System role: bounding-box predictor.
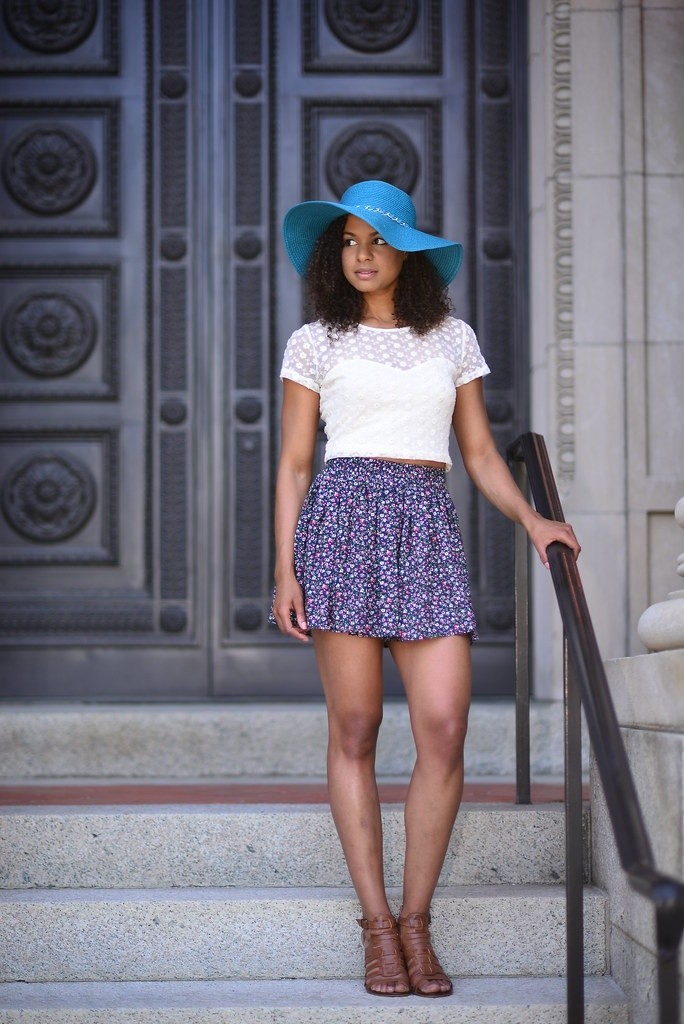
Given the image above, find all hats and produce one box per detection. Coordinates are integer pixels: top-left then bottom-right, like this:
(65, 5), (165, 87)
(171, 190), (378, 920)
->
(281, 179), (464, 289)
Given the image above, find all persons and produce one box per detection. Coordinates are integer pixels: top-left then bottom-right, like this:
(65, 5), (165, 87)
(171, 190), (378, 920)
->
(265, 180), (584, 1000)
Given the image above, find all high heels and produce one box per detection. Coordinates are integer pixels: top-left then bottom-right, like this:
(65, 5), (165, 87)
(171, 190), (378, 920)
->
(396, 906), (453, 998)
(355, 913), (411, 996)
